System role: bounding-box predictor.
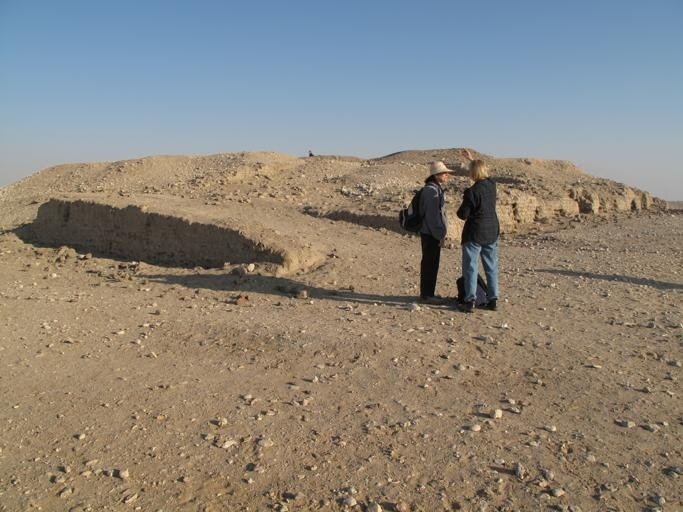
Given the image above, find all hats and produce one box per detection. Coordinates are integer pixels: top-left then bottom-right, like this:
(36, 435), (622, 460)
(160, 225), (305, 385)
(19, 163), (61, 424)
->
(424, 160), (456, 183)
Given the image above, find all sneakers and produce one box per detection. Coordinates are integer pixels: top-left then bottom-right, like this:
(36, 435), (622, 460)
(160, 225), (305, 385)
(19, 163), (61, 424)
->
(420, 296), (442, 305)
(485, 300), (496, 309)
(461, 300), (476, 312)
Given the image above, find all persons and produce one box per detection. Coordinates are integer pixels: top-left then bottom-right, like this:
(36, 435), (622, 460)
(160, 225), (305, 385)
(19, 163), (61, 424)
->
(418, 160), (459, 305)
(454, 146), (501, 313)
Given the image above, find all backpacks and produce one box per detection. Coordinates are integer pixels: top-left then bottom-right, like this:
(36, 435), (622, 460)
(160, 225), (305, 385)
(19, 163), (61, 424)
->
(456, 272), (488, 306)
(399, 184), (440, 233)
